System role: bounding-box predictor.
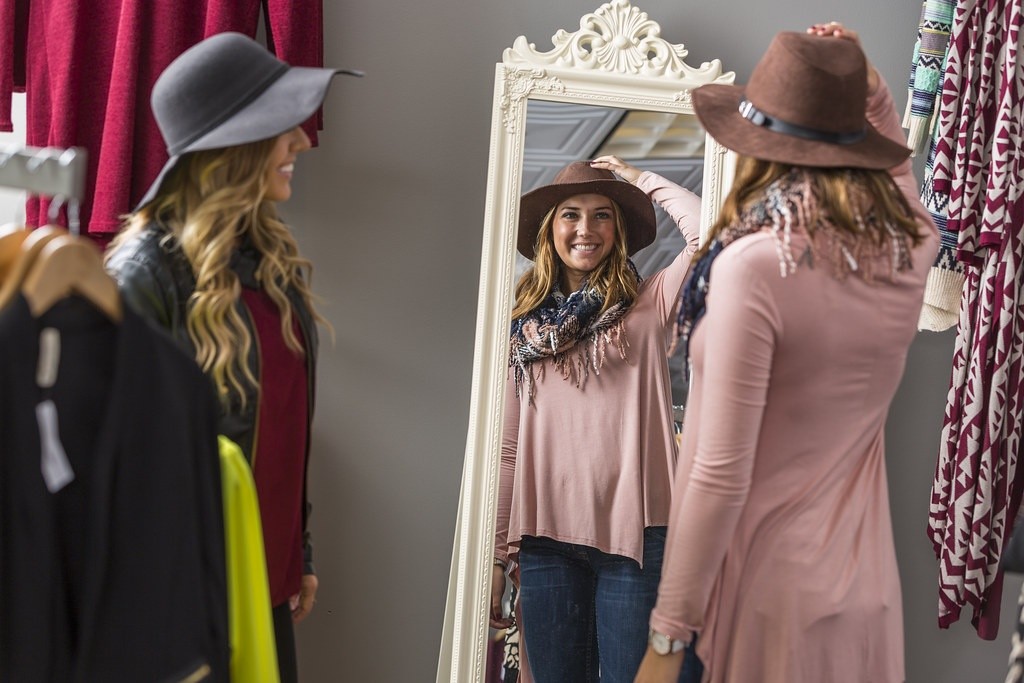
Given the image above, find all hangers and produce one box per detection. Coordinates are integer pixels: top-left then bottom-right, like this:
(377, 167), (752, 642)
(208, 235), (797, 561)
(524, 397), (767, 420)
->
(0, 147), (202, 393)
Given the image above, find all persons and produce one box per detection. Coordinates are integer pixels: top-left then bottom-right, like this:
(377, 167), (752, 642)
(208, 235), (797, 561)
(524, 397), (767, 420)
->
(102, 32), (366, 683)
(488, 155), (701, 683)
(633, 21), (940, 683)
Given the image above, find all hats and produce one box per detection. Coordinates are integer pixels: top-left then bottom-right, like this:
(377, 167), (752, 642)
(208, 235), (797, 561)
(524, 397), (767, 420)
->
(691, 30), (916, 171)
(516, 162), (659, 263)
(131, 32), (364, 210)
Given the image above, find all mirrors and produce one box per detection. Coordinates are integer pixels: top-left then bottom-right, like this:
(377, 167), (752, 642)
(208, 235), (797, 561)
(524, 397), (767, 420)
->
(434, 0), (737, 683)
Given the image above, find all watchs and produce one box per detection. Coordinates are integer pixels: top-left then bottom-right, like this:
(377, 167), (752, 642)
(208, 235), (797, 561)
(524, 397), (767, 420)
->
(647, 630), (684, 655)
(494, 559), (507, 570)
